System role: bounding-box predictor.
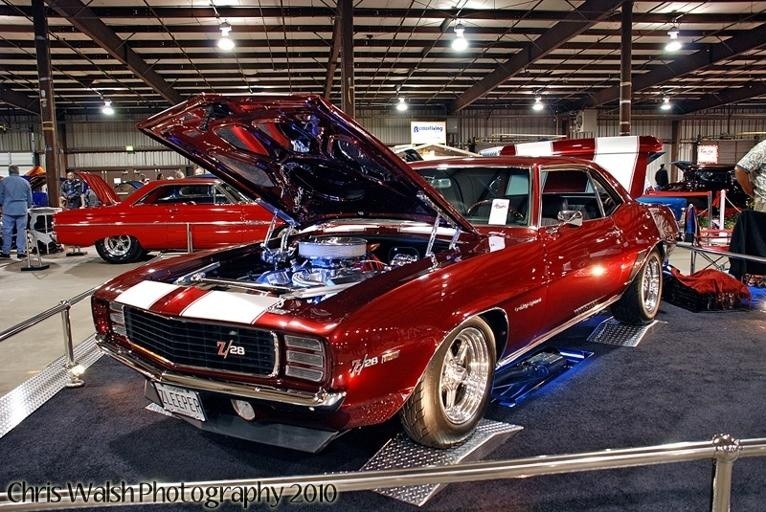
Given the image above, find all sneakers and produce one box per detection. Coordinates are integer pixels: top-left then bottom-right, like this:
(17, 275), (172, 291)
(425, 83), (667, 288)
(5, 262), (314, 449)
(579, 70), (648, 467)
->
(17, 254), (27, 258)
(0, 254), (10, 258)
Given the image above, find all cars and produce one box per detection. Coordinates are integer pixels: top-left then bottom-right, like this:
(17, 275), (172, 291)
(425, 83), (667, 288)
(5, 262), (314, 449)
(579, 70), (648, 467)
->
(660, 159), (753, 209)
(89, 91), (680, 451)
(51, 170), (301, 265)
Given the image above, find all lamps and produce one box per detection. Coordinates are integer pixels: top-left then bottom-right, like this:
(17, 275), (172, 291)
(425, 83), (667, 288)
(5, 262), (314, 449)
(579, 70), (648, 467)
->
(532, 96), (544, 112)
(664, 26), (682, 51)
(215, 22), (236, 50)
(101, 98), (114, 115)
(452, 24), (469, 49)
(660, 96), (671, 110)
(396, 97), (409, 112)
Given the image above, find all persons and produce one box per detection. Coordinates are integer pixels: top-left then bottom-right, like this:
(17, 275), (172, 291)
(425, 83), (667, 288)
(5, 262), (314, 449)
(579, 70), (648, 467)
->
(156, 174), (164, 181)
(733, 137), (766, 216)
(174, 169), (185, 180)
(139, 173), (149, 185)
(655, 163), (669, 188)
(60, 172), (87, 210)
(0, 164), (33, 260)
